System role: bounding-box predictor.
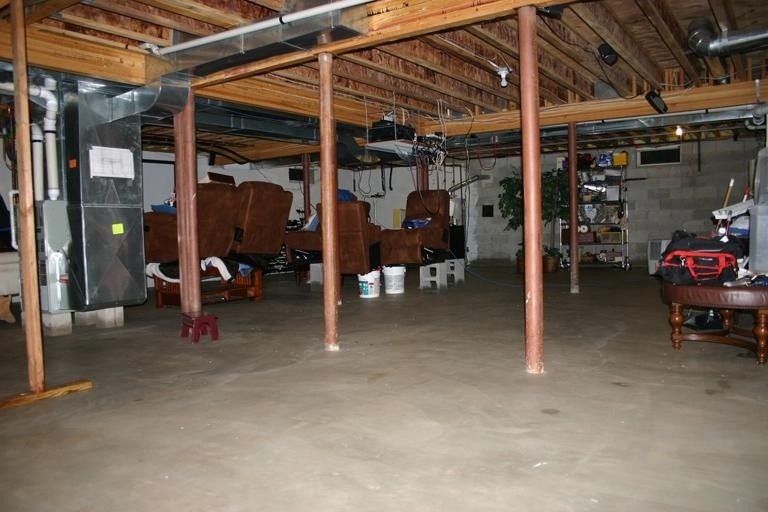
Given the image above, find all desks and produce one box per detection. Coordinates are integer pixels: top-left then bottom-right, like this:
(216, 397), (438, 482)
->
(662, 282), (768, 366)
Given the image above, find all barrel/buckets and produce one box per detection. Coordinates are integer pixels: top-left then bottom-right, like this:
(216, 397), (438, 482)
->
(381, 264), (406, 295)
(357, 269), (383, 298)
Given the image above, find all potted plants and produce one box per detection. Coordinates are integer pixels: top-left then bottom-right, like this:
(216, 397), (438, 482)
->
(497, 169), (582, 274)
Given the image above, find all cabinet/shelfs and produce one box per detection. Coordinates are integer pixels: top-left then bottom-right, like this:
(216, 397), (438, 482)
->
(560, 164), (632, 271)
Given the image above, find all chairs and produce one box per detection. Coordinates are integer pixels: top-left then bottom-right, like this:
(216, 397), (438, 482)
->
(146, 180), (238, 265)
(233, 180), (293, 259)
(374, 187), (450, 267)
(318, 199), (374, 274)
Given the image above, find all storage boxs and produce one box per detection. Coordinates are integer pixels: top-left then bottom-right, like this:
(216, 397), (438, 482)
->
(613, 153), (629, 166)
(579, 231), (626, 244)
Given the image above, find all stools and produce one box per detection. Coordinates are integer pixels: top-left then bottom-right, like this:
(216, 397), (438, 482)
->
(181, 310), (219, 344)
(419, 258), (465, 289)
